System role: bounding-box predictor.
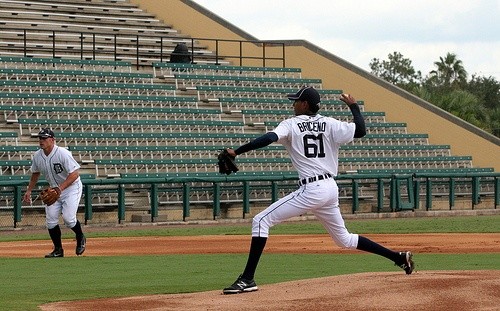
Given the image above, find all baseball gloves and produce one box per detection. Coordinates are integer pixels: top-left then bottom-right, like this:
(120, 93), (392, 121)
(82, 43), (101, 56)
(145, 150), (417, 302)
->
(217, 149), (240, 175)
(40, 187), (62, 207)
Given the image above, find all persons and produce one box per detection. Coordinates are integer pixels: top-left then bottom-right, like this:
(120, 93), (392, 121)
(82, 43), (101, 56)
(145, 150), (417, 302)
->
(23, 129), (86, 257)
(221, 87), (415, 294)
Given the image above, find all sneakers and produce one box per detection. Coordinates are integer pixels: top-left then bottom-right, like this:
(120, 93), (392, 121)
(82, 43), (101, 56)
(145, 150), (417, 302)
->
(75, 236), (86, 255)
(398, 251), (414, 274)
(44, 248), (63, 258)
(223, 273), (258, 293)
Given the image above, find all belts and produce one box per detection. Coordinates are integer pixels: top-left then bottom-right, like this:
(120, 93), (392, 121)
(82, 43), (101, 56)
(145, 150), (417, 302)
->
(299, 173), (332, 186)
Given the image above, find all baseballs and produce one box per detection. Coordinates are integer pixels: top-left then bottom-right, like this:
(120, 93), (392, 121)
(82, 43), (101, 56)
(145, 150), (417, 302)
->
(340, 94), (348, 99)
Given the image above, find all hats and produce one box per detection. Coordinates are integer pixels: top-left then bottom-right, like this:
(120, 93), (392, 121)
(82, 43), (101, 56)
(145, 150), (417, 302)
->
(31, 129), (54, 138)
(288, 87), (320, 103)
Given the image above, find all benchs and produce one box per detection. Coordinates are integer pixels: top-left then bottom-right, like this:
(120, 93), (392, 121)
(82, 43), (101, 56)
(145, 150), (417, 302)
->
(1, 0), (499, 205)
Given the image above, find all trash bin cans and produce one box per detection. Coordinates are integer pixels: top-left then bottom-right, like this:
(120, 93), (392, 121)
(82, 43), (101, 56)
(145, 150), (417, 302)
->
(169, 43), (192, 72)
